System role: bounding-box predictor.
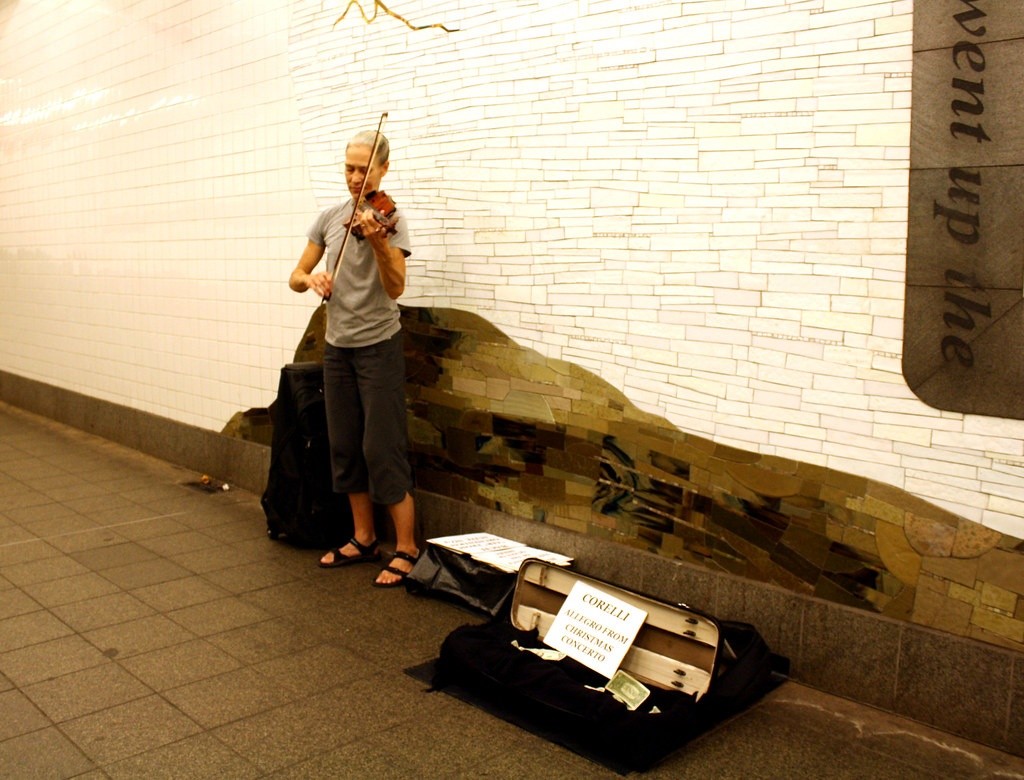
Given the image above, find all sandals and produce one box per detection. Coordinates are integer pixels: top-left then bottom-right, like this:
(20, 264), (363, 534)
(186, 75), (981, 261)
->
(318, 538), (381, 567)
(373, 550), (419, 587)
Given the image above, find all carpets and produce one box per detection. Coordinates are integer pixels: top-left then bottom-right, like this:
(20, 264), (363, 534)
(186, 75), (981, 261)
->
(404, 657), (787, 776)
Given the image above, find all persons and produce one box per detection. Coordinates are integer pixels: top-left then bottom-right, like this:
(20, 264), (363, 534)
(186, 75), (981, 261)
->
(287, 130), (423, 585)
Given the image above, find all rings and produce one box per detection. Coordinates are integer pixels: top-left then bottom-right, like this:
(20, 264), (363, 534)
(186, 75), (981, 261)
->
(361, 224), (366, 229)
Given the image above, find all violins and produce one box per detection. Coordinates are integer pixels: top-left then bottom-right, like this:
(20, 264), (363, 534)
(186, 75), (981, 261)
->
(343, 190), (401, 240)
